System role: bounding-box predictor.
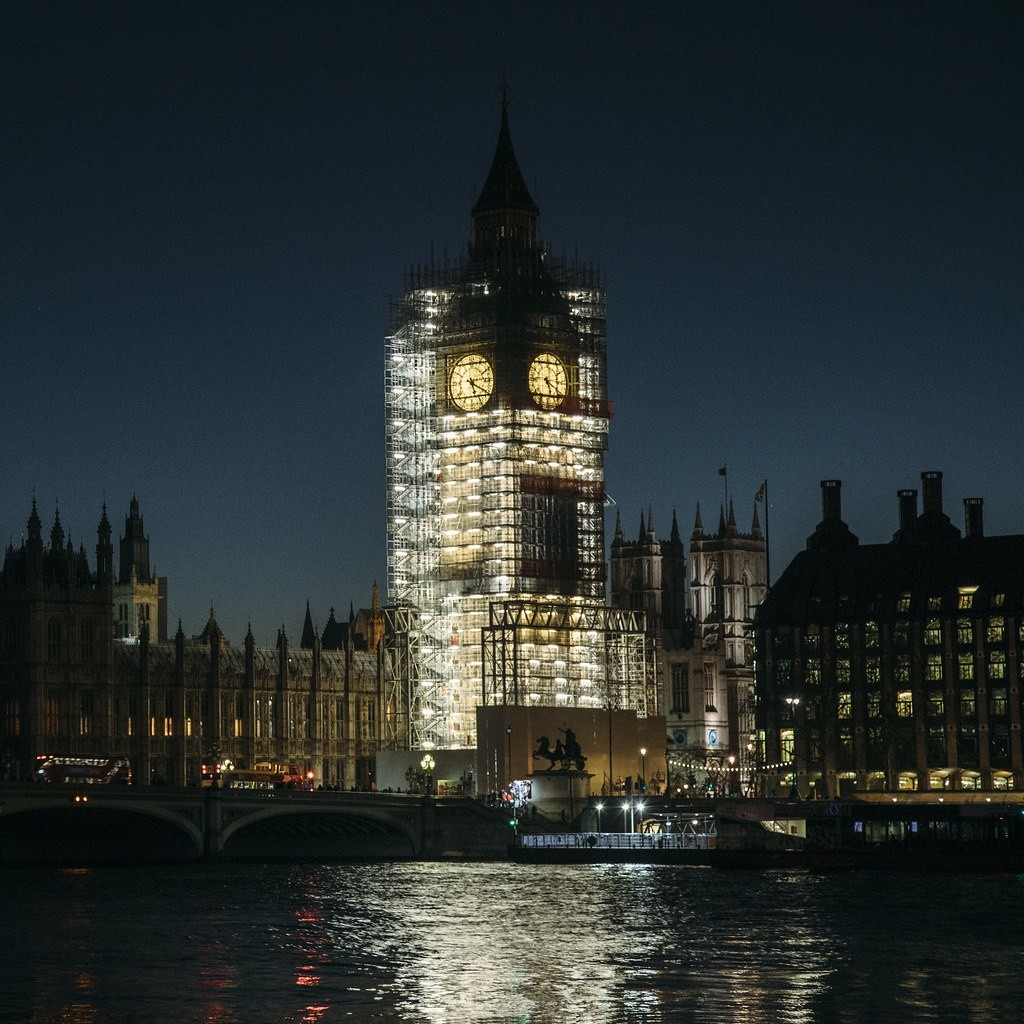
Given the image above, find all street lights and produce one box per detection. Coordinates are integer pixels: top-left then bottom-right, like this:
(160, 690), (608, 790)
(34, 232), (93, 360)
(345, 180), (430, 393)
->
(747, 743), (753, 768)
(785, 696), (799, 785)
(729, 754), (736, 794)
(421, 755), (435, 796)
(640, 747), (647, 781)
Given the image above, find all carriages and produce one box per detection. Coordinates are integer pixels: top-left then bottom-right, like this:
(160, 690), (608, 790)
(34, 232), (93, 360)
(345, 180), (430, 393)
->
(532, 736), (587, 771)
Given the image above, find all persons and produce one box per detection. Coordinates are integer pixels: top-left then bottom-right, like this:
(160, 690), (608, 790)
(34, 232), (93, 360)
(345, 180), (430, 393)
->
(554, 739), (565, 753)
(316, 784), (370, 792)
(489, 788), (517, 808)
(380, 787), (402, 793)
(524, 804), (529, 822)
(561, 808), (569, 824)
(532, 804), (537, 822)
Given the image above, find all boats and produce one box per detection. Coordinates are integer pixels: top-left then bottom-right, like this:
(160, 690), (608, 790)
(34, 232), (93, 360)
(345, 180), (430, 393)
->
(512, 814), (806, 866)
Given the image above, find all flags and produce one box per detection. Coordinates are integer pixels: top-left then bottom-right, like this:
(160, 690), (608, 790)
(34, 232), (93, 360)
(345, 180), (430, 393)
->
(719, 467), (726, 477)
(755, 483), (765, 502)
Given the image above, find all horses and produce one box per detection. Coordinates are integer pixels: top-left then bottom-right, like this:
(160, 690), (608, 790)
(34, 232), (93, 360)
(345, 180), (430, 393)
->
(533, 736), (564, 771)
